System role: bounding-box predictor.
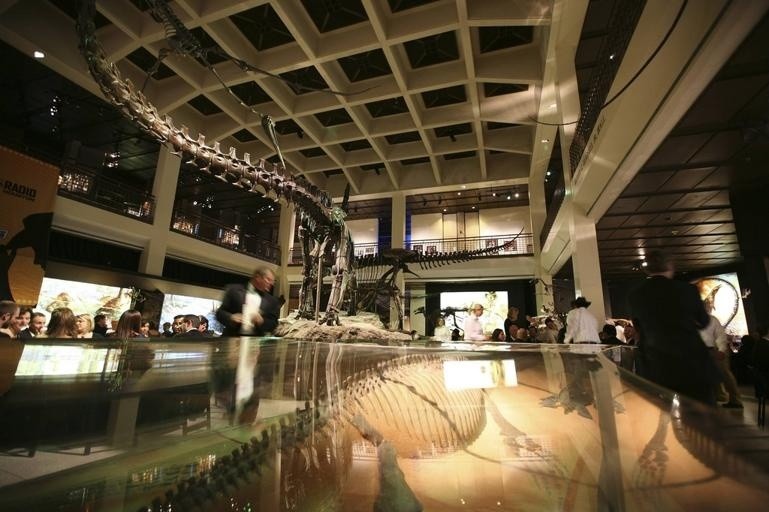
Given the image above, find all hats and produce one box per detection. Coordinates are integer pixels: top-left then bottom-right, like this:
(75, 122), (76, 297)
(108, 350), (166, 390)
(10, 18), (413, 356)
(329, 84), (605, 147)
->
(571, 297), (591, 309)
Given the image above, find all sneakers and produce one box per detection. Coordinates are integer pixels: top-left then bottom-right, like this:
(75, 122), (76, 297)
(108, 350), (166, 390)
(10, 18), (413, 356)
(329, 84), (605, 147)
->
(721, 402), (744, 411)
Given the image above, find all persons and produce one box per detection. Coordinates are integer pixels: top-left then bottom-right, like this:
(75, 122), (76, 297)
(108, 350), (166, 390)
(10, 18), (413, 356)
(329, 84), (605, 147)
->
(0, 299), (215, 339)
(46, 292), (72, 313)
(630, 251), (769, 408)
(215, 265), (281, 338)
(433, 296), (639, 346)
